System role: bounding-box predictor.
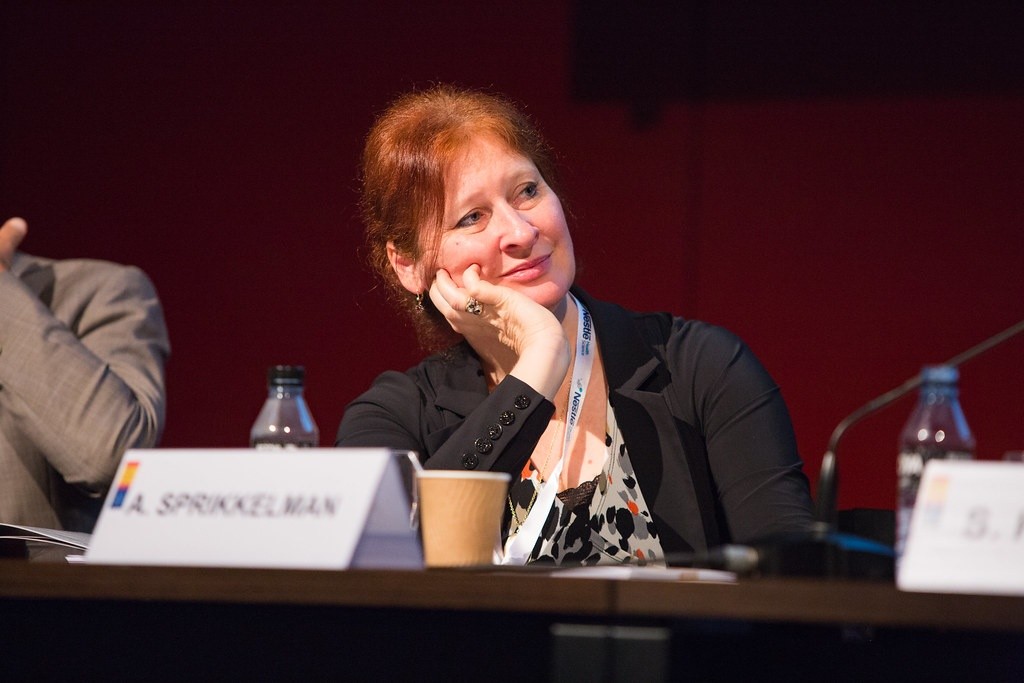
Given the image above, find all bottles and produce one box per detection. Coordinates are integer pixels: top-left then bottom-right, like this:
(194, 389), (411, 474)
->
(894, 366), (976, 579)
(250, 363), (319, 449)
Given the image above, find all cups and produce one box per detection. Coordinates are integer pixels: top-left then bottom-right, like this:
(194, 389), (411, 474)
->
(391, 451), (423, 531)
(418, 471), (512, 568)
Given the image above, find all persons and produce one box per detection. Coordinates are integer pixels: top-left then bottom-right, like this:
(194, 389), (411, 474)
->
(334, 87), (814, 564)
(0, 217), (171, 561)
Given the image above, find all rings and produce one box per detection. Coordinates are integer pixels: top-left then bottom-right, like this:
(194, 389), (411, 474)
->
(464, 296), (482, 316)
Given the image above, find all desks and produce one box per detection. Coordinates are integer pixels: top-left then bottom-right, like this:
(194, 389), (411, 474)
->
(618, 571), (1024, 683)
(0, 560), (619, 683)
